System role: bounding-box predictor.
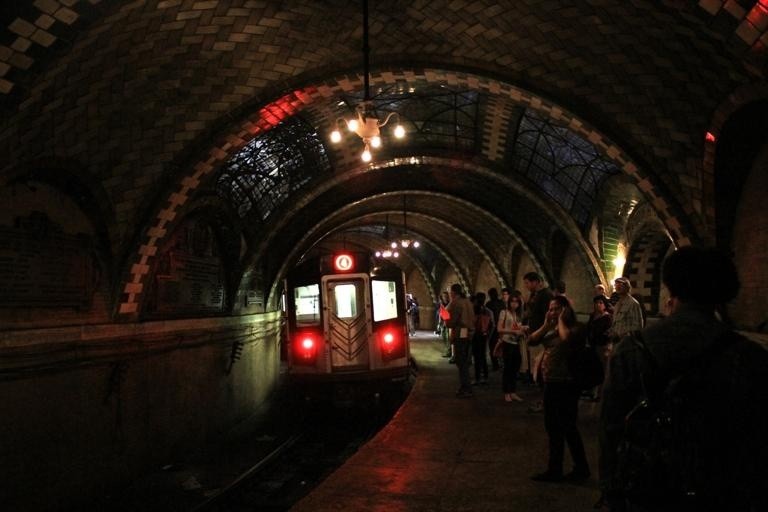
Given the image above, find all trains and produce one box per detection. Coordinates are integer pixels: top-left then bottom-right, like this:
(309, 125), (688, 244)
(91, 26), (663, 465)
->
(284, 252), (410, 410)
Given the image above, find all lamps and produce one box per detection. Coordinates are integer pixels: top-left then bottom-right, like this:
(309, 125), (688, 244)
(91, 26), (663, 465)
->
(329, 1), (406, 164)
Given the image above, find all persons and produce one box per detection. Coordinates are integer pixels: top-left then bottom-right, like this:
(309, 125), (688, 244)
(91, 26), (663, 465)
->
(413, 297), (420, 329)
(524, 296), (594, 486)
(406, 296), (416, 335)
(597, 240), (767, 510)
(437, 269), (652, 414)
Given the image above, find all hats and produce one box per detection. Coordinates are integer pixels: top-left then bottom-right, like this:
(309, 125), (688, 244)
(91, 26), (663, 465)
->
(616, 276), (632, 288)
(661, 247), (744, 305)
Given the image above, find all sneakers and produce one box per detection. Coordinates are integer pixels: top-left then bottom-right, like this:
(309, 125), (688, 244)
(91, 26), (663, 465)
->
(503, 391), (523, 403)
(528, 468), (592, 483)
(579, 389), (601, 401)
(456, 378), (490, 398)
(527, 399), (544, 412)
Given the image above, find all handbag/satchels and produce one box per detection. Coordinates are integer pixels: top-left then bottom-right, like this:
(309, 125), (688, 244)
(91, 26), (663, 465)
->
(608, 329), (706, 503)
